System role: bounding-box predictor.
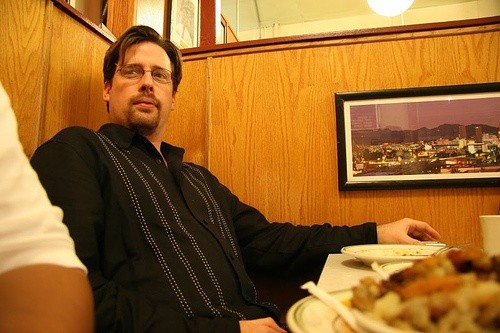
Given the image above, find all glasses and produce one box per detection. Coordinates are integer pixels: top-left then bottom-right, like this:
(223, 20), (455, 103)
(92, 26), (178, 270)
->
(111, 60), (175, 85)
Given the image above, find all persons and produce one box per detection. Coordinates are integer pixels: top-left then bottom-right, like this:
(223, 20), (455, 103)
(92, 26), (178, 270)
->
(32, 26), (443, 333)
(0, 81), (93, 333)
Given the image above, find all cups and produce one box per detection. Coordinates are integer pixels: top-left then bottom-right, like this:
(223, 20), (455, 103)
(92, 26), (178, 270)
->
(478, 215), (500, 255)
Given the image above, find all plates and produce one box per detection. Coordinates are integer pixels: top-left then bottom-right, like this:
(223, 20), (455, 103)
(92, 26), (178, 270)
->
(380, 261), (414, 274)
(286, 287), (374, 333)
(340, 244), (446, 268)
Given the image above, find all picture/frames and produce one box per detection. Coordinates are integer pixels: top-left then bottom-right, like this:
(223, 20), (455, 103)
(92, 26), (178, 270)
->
(335, 83), (500, 192)
